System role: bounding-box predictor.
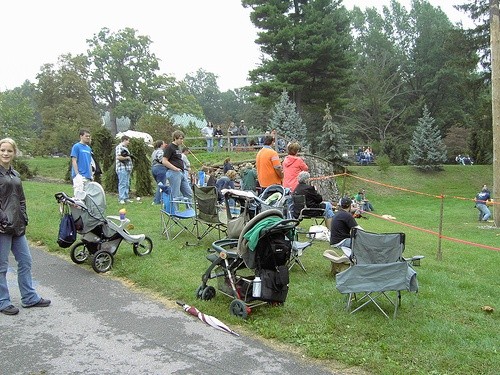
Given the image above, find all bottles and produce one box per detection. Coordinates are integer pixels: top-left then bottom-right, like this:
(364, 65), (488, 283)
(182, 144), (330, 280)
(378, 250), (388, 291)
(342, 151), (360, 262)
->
(252, 277), (261, 297)
(119, 209), (126, 220)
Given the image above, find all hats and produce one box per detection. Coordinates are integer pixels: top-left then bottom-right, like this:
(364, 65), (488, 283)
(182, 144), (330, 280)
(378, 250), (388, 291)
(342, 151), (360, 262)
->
(341, 197), (353, 207)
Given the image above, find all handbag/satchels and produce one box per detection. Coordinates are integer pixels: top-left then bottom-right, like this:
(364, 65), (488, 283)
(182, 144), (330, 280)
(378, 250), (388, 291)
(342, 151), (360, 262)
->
(254, 269), (288, 303)
(57, 202), (76, 247)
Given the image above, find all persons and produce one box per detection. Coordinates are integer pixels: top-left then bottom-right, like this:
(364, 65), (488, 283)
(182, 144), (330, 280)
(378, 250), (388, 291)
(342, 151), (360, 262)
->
(357, 146), (373, 165)
(330, 198), (364, 248)
(115, 135), (133, 204)
(196, 158), (260, 213)
(256, 136), (284, 194)
(70, 129), (102, 200)
(456, 154), (473, 166)
(0, 138), (51, 315)
(282, 143), (309, 193)
(292, 171), (335, 231)
(474, 185), (491, 222)
(182, 148), (191, 181)
(152, 140), (166, 205)
(162, 130), (194, 221)
(201, 119), (305, 152)
(338, 187), (375, 218)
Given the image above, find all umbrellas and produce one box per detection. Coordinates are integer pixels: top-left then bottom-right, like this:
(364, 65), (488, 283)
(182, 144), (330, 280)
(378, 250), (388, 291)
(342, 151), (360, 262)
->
(176, 301), (239, 337)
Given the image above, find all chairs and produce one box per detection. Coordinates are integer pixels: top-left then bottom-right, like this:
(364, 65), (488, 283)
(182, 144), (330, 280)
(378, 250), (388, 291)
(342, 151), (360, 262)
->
(287, 231), (316, 274)
(192, 184), (229, 242)
(293, 194), (327, 226)
(158, 182), (199, 241)
(346, 228), (406, 322)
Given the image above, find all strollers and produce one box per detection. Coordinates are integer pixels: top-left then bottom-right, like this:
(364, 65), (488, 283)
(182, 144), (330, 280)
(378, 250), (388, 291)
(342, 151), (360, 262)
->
(55, 181), (153, 274)
(223, 183), (293, 249)
(196, 209), (301, 319)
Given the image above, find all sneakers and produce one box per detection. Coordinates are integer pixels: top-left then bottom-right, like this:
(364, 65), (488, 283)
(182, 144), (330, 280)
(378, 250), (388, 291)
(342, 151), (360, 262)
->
(2, 305), (19, 315)
(25, 298), (51, 308)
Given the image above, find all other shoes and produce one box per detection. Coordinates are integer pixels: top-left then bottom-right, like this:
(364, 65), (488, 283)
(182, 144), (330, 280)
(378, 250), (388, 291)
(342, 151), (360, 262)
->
(128, 199), (134, 203)
(120, 199), (125, 204)
